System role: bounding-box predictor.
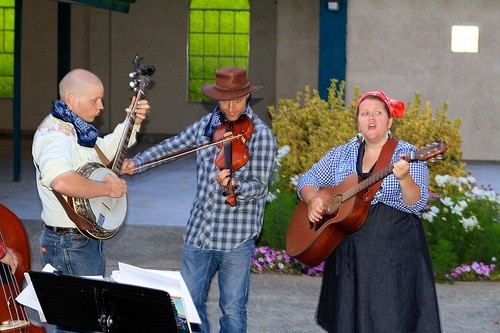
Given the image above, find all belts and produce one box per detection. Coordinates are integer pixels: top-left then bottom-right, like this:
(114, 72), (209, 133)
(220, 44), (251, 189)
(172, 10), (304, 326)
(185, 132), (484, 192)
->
(45, 224), (82, 235)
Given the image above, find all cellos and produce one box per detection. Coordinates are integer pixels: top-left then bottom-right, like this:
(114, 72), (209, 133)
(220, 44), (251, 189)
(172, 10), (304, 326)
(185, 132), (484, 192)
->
(0, 202), (48, 333)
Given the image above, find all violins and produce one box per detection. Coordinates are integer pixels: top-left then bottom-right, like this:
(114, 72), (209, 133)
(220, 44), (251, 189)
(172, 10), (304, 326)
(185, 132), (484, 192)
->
(212, 114), (254, 206)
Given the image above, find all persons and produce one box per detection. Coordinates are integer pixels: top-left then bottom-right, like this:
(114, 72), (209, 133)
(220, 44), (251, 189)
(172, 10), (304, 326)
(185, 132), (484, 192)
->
(121, 68), (275, 333)
(31, 68), (150, 280)
(297, 90), (442, 333)
(0, 244), (20, 275)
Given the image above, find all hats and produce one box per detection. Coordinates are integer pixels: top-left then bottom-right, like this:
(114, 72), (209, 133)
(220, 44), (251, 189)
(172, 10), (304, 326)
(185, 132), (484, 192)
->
(201, 68), (262, 101)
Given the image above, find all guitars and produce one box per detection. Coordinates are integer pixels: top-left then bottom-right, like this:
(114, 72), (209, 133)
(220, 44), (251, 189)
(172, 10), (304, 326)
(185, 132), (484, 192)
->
(67, 53), (155, 241)
(284, 140), (448, 267)
